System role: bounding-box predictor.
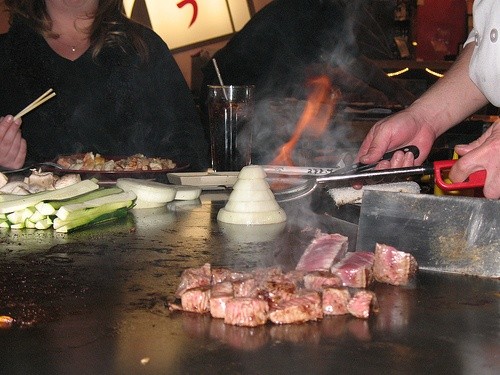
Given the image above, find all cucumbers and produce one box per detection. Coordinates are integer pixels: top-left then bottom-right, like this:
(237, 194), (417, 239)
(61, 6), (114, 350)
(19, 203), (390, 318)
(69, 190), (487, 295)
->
(0, 180), (138, 234)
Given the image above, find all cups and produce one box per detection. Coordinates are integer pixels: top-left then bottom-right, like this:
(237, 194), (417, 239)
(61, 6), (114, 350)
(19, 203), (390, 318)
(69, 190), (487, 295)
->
(208, 85), (256, 173)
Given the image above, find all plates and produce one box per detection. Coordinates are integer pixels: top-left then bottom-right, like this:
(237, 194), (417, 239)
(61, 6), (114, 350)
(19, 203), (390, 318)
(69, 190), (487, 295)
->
(32, 154), (190, 184)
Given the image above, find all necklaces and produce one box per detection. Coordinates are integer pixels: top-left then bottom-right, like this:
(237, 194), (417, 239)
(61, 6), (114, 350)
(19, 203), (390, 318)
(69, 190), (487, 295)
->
(54, 36), (87, 53)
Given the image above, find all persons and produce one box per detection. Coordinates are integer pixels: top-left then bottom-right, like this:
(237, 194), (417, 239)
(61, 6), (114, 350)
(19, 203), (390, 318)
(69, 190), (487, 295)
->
(0, 1), (211, 172)
(202, 1), (418, 118)
(352, 1), (500, 200)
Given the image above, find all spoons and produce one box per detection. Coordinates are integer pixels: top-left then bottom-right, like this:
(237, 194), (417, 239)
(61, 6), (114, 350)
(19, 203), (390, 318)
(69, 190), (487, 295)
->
(0, 162), (65, 173)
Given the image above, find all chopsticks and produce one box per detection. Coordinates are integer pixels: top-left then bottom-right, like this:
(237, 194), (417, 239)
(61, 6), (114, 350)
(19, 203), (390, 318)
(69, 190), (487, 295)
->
(13, 89), (56, 121)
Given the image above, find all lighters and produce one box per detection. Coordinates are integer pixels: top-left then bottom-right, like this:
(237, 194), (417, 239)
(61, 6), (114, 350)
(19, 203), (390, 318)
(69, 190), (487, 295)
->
(317, 158), (486, 191)
